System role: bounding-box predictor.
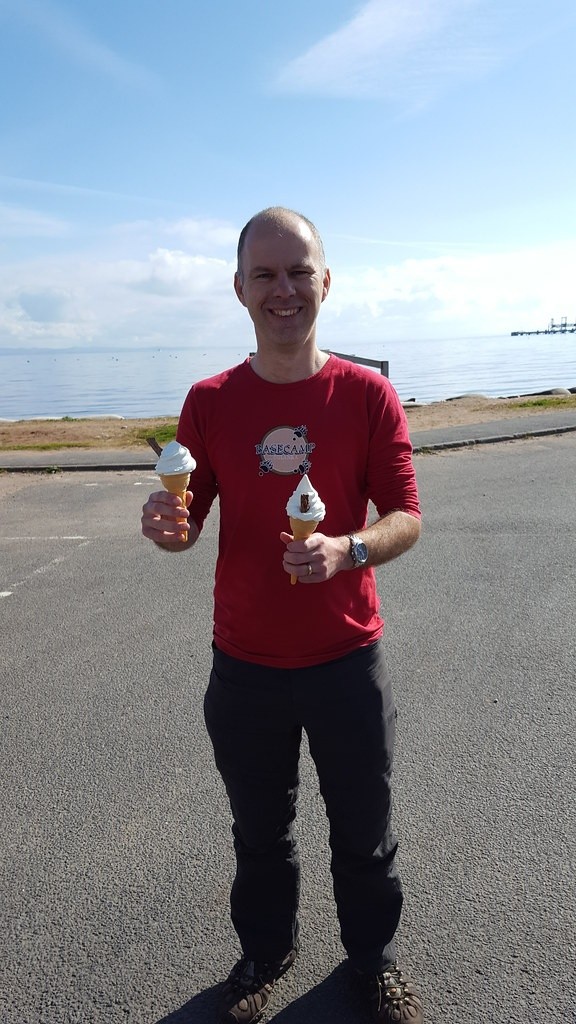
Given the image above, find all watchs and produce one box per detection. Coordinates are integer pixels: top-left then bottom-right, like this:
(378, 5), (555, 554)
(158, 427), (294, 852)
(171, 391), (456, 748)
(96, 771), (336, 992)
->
(346, 533), (368, 570)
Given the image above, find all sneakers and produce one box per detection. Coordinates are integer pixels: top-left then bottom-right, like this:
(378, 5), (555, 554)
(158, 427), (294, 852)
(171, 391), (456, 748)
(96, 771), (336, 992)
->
(218, 939), (300, 1024)
(349, 955), (423, 1024)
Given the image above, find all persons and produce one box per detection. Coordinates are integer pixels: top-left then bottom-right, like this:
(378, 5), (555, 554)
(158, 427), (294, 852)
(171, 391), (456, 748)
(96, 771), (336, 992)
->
(141, 206), (424, 1024)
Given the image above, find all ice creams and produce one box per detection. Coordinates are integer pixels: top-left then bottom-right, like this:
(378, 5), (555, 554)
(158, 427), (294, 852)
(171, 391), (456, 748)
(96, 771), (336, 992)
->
(285, 473), (325, 585)
(146, 436), (196, 542)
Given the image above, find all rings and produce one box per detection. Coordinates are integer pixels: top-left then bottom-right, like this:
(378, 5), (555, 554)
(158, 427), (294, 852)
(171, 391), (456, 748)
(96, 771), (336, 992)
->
(308, 564), (313, 575)
